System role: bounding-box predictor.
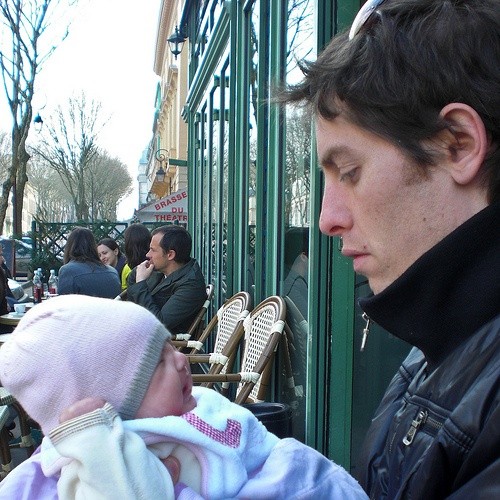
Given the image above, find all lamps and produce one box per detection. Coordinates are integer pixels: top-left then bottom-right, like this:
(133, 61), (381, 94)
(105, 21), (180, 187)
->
(167, 23), (188, 60)
(156, 167), (166, 182)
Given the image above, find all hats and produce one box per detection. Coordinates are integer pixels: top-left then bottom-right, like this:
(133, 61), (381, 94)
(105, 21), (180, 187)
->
(1, 294), (171, 436)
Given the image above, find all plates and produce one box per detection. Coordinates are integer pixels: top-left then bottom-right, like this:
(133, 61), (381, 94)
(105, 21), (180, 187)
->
(9, 312), (25, 317)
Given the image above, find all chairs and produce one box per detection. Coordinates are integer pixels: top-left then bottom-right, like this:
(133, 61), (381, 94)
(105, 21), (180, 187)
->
(0, 284), (287, 476)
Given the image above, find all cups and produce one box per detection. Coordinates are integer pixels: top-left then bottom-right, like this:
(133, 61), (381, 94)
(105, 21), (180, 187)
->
(14, 304), (26, 314)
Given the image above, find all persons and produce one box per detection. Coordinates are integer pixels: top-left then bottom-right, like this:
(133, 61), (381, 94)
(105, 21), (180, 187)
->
(127, 225), (206, 334)
(121, 225), (152, 302)
(97, 238), (132, 292)
(260, 0), (500, 500)
(57, 226), (121, 300)
(0, 295), (370, 500)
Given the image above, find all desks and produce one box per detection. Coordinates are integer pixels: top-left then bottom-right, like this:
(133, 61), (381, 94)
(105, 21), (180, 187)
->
(0, 312), (25, 325)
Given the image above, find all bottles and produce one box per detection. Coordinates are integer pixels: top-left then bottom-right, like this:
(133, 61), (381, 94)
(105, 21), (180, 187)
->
(48, 270), (58, 295)
(34, 271), (43, 304)
(37, 267), (44, 295)
